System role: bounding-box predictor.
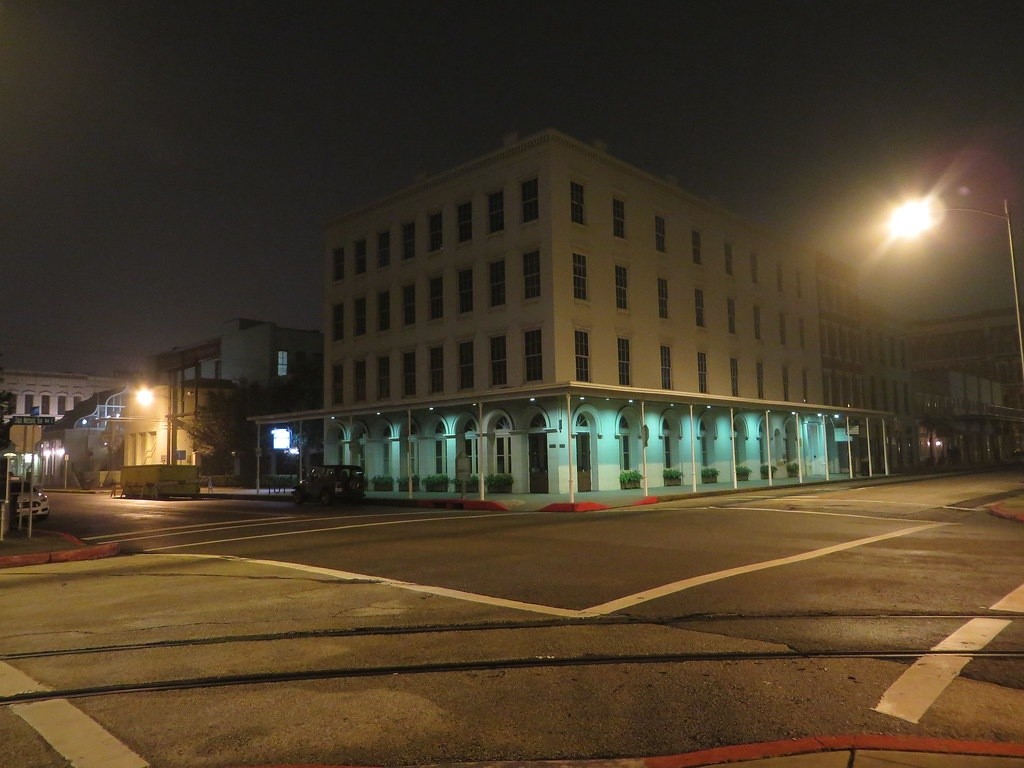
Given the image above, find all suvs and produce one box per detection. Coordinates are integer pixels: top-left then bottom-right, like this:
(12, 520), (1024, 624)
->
(292, 464), (366, 509)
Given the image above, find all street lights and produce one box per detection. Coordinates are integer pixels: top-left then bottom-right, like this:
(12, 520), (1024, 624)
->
(137, 386), (172, 465)
(892, 197), (1024, 373)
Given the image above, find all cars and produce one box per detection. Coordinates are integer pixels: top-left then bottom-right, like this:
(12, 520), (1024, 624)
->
(0, 479), (50, 522)
